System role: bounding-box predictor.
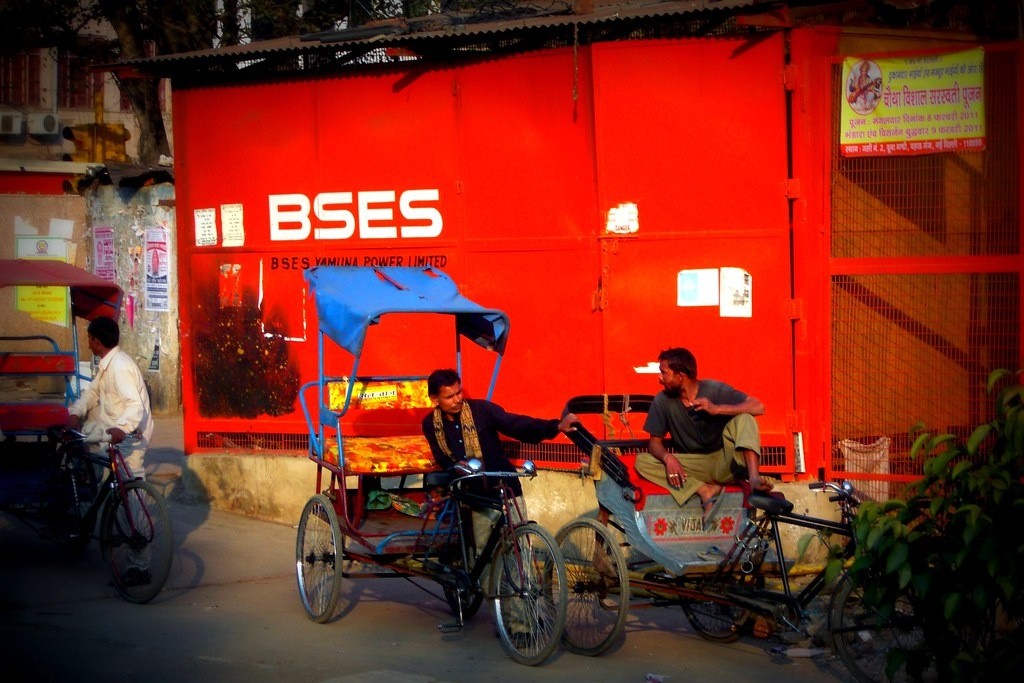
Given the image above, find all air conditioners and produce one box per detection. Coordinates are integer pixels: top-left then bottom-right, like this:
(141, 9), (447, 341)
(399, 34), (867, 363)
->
(27, 113), (59, 134)
(0, 111), (21, 134)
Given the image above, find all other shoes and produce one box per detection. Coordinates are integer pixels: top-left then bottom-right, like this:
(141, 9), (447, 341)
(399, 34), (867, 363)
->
(111, 566), (154, 591)
(496, 620), (536, 647)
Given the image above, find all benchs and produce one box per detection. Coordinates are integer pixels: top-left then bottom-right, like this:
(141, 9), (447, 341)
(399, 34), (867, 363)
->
(616, 455), (754, 511)
(324, 380), (444, 529)
(0, 352), (77, 435)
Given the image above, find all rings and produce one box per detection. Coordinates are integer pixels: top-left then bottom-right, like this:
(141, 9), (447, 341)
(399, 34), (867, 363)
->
(673, 473), (678, 476)
(669, 474), (673, 477)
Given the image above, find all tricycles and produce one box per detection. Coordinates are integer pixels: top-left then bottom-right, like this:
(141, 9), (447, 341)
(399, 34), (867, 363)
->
(544, 394), (931, 683)
(294, 263), (569, 668)
(0, 260), (174, 605)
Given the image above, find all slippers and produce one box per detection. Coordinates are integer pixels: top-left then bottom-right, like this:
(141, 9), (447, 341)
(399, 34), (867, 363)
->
(701, 484), (727, 523)
(749, 482), (772, 494)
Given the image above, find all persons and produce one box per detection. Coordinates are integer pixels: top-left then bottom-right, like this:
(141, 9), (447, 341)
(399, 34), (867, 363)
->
(422, 370), (579, 647)
(634, 348), (774, 529)
(66, 317), (154, 588)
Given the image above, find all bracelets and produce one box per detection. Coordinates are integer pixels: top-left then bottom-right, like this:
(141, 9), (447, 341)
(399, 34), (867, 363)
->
(664, 453), (671, 461)
